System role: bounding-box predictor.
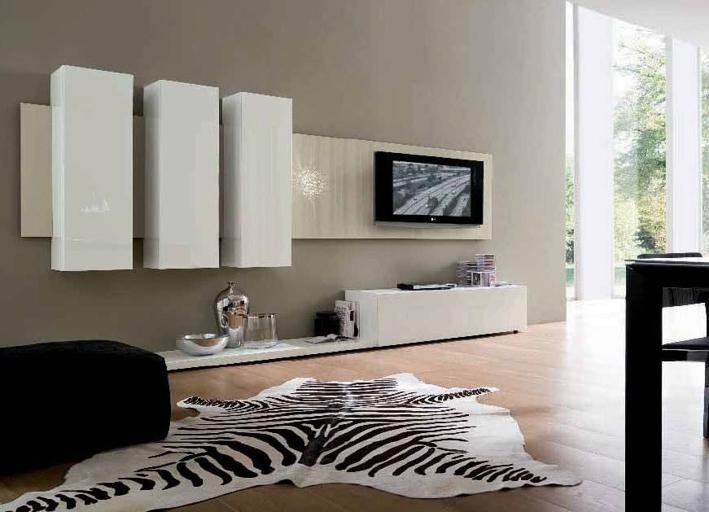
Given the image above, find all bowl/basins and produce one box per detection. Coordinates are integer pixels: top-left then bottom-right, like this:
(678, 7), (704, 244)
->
(176, 332), (232, 355)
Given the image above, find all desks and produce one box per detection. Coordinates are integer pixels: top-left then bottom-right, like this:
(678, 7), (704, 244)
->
(626, 252), (709, 512)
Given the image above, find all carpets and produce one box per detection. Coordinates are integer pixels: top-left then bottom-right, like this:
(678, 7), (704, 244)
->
(0, 372), (582, 512)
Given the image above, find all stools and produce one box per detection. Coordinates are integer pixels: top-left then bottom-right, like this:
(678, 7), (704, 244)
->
(0, 340), (171, 474)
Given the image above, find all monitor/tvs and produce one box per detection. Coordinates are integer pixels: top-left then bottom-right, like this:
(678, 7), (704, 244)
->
(374, 149), (484, 228)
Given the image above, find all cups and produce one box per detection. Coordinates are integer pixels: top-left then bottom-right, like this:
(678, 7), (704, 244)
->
(243, 310), (280, 350)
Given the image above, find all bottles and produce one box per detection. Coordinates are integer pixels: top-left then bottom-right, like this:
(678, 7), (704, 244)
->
(214, 278), (250, 347)
